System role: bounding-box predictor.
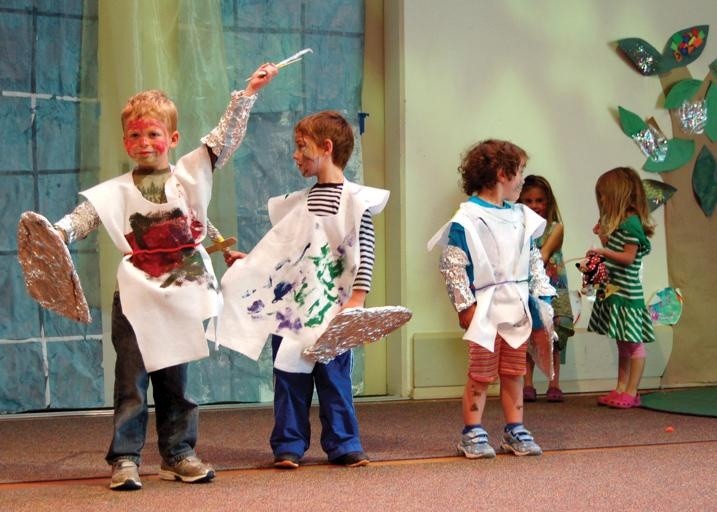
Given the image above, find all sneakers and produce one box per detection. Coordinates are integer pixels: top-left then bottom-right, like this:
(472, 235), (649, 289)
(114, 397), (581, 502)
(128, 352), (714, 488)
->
(272, 453), (299, 468)
(109, 459), (142, 489)
(160, 456), (216, 483)
(331, 451), (369, 467)
(457, 427), (496, 459)
(499, 424), (543, 456)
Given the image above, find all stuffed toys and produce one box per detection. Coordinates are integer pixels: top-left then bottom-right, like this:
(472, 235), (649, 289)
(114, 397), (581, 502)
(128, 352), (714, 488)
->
(575, 254), (610, 302)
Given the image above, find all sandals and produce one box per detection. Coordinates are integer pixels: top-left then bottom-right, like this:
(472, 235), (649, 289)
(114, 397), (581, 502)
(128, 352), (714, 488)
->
(546, 387), (563, 401)
(607, 392), (641, 409)
(597, 390), (624, 405)
(523, 387), (536, 400)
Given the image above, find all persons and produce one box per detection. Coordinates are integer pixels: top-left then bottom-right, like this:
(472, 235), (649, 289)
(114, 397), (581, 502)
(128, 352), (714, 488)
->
(517, 175), (575, 402)
(52, 62), (277, 491)
(224, 111), (392, 468)
(439, 138), (563, 459)
(588, 167), (656, 408)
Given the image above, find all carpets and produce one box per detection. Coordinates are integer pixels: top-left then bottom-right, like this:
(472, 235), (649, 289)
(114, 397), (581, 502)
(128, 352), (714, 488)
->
(1, 443), (717, 512)
(639, 387), (717, 418)
(1, 388), (716, 483)
